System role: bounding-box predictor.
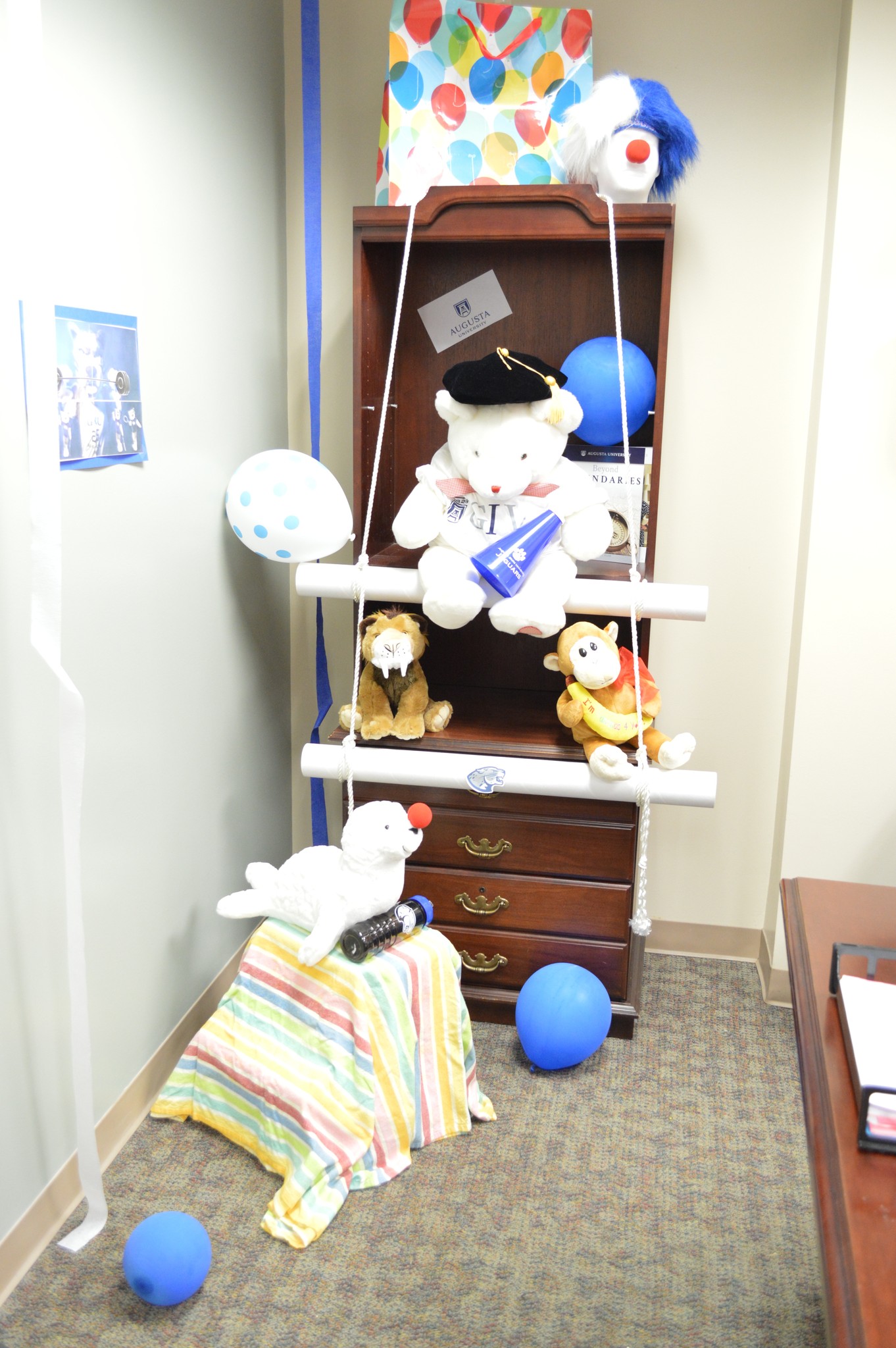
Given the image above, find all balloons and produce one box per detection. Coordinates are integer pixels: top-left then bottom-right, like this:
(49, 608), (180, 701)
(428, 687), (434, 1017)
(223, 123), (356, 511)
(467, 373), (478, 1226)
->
(123, 1210), (211, 1305)
(547, 337), (659, 447)
(516, 963), (612, 1069)
(226, 448), (354, 564)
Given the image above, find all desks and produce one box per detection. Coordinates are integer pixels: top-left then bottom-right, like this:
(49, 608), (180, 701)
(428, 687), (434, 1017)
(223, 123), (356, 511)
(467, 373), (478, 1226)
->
(779, 877), (896, 1347)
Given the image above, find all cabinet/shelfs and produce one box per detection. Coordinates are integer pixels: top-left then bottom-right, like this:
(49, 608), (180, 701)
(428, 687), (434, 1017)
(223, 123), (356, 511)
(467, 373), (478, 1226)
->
(348, 184), (677, 1036)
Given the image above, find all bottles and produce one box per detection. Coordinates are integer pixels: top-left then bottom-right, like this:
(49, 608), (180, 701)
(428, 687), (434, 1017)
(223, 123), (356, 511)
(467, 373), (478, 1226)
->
(340, 895), (433, 965)
(470, 509), (563, 598)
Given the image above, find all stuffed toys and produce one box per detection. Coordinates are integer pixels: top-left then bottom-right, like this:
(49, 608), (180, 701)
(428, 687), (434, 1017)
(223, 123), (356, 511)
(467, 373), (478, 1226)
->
(388, 348), (608, 638)
(214, 800), (433, 968)
(337, 609), (454, 743)
(542, 620), (696, 782)
